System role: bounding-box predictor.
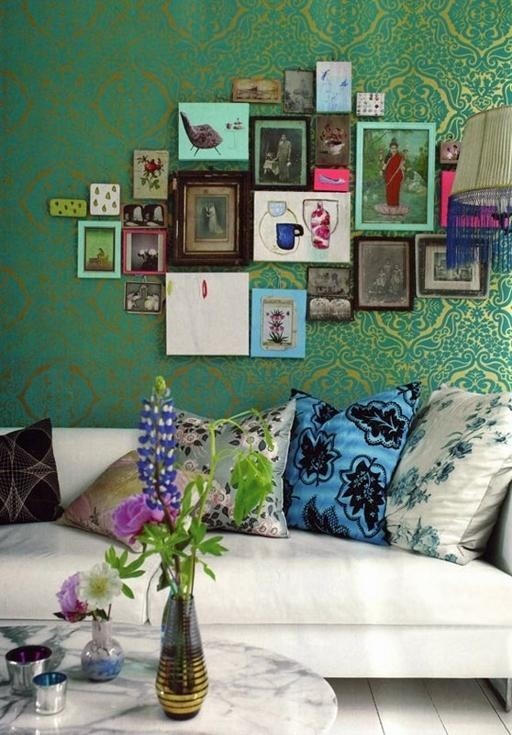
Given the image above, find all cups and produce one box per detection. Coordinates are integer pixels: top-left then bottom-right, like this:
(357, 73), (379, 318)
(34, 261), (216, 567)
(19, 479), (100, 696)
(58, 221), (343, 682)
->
(5, 644), (53, 693)
(31, 672), (68, 715)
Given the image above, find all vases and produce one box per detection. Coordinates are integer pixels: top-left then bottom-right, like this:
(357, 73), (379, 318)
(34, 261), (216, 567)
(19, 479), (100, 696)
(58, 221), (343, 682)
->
(82, 621), (125, 680)
(155, 594), (208, 719)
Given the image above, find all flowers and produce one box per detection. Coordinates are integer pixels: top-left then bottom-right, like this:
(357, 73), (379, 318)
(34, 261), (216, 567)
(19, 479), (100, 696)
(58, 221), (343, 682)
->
(112, 376), (274, 595)
(53, 543), (147, 621)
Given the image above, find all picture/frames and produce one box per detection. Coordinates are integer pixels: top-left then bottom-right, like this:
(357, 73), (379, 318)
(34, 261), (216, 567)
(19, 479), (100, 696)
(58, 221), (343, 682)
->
(123, 228), (166, 274)
(89, 183), (121, 215)
(415, 235), (491, 298)
(440, 142), (463, 165)
(354, 236), (416, 312)
(316, 61), (352, 114)
(177, 101), (251, 161)
(254, 192), (351, 264)
(356, 121), (436, 231)
(304, 263), (355, 322)
(133, 149), (169, 199)
(251, 289), (307, 360)
(313, 167), (350, 191)
(125, 281), (163, 315)
(166, 271), (250, 356)
(357, 92), (385, 117)
(171, 172), (253, 266)
(251, 115), (311, 188)
(78, 220), (122, 279)
(440, 169), (509, 229)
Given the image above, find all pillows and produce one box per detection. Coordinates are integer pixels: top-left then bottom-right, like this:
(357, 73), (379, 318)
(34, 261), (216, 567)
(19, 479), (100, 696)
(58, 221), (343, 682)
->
(382, 382), (511, 564)
(283, 381), (422, 549)
(0, 415), (64, 526)
(171, 402), (296, 539)
(55, 449), (229, 554)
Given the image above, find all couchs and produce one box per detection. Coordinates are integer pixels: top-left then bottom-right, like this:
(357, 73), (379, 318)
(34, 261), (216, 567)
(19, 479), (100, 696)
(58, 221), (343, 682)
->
(0, 427), (512, 711)
(180, 111), (223, 156)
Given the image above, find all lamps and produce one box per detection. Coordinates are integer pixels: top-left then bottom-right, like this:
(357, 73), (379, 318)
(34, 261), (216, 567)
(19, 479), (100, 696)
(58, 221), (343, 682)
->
(448, 106), (511, 273)
